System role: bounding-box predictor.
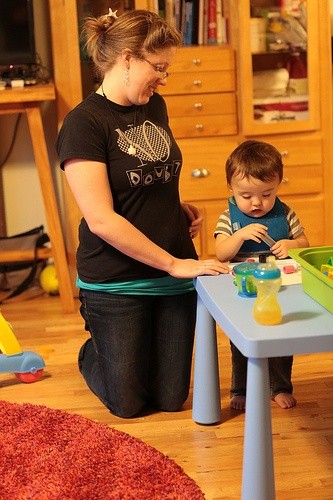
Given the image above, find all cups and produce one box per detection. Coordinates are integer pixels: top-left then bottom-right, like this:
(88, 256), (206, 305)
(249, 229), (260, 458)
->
(250, 18), (267, 54)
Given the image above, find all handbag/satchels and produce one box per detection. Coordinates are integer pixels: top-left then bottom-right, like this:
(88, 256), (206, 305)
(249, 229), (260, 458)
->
(0, 226), (46, 290)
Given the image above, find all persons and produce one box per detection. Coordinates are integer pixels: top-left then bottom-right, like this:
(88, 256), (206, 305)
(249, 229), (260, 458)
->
(54, 8), (230, 420)
(211, 140), (310, 410)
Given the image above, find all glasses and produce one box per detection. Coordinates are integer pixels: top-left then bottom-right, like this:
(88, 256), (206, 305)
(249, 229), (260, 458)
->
(137, 54), (169, 80)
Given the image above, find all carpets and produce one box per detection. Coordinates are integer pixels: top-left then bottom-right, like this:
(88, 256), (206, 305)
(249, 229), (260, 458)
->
(0, 400), (212, 500)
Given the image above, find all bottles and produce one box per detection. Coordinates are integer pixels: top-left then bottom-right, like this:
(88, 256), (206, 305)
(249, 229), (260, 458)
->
(266, 12), (289, 52)
(253, 263), (283, 325)
(233, 259), (258, 297)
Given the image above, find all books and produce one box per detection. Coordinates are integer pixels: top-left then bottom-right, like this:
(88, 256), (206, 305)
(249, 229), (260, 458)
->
(134, 0), (229, 45)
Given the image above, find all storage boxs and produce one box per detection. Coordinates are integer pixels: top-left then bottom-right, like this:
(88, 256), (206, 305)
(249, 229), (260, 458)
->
(288, 246), (333, 315)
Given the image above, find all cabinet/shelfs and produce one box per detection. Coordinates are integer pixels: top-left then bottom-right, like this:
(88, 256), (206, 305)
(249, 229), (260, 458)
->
(120, 1), (333, 254)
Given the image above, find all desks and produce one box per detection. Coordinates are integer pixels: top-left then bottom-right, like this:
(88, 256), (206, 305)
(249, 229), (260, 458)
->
(0, 80), (75, 313)
(190, 270), (333, 500)
